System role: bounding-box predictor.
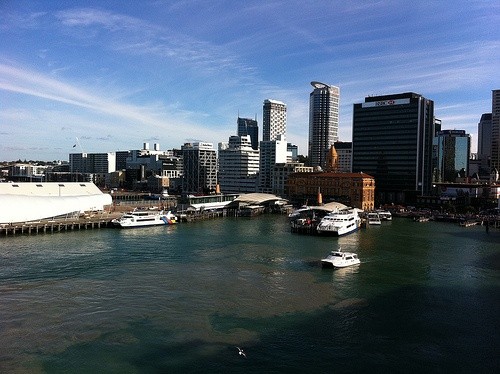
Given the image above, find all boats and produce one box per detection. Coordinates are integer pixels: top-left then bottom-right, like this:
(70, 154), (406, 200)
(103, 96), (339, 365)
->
(316, 208), (361, 236)
(118, 211), (178, 227)
(321, 251), (361, 268)
(367, 210), (393, 225)
